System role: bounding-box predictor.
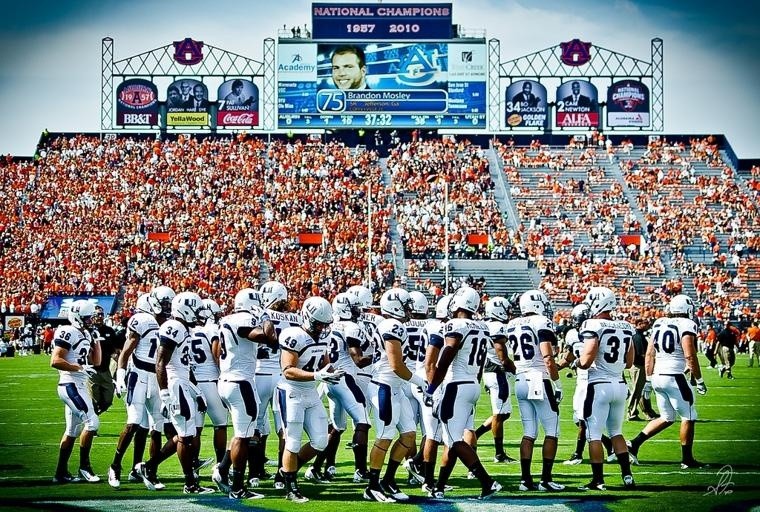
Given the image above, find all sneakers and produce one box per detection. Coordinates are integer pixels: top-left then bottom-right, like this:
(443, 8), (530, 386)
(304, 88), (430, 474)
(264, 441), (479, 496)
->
(468, 470), (475, 479)
(401, 458), (453, 498)
(537, 480), (566, 491)
(563, 453), (583, 465)
(577, 482), (606, 491)
(623, 475), (635, 487)
(304, 464), (336, 484)
(353, 468), (409, 503)
(182, 457), (309, 504)
(494, 453), (519, 464)
(681, 460), (711, 470)
(706, 364), (735, 379)
(629, 414), (660, 421)
(478, 480), (503, 500)
(606, 439), (640, 466)
(518, 480), (538, 491)
(53, 462), (165, 491)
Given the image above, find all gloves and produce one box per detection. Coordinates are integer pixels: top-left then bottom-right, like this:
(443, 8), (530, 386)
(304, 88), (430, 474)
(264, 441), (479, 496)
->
(314, 364), (346, 385)
(160, 389), (175, 419)
(194, 384), (207, 414)
(91, 325), (101, 343)
(116, 368), (127, 398)
(554, 378), (563, 404)
(641, 381), (655, 400)
(408, 374), (433, 407)
(79, 365), (98, 378)
(696, 381), (707, 395)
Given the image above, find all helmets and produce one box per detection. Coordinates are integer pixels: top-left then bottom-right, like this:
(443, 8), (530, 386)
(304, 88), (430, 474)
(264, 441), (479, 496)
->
(234, 281), (288, 312)
(136, 286), (221, 324)
(302, 296), (334, 339)
(571, 303), (591, 325)
(485, 296), (512, 321)
(67, 299), (95, 329)
(380, 288), (428, 319)
(436, 287), (481, 318)
(669, 295), (695, 320)
(332, 285), (373, 320)
(582, 287), (616, 316)
(519, 290), (550, 315)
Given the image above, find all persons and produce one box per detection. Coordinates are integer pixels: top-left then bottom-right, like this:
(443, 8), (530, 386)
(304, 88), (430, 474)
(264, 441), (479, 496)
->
(331, 45), (370, 89)
(297, 26), (300, 37)
(186, 85), (209, 111)
(224, 80), (256, 108)
(291, 27), (295, 38)
(0, 128), (756, 504)
(168, 87), (186, 109)
(179, 81), (194, 102)
(562, 82), (590, 108)
(512, 82), (541, 107)
